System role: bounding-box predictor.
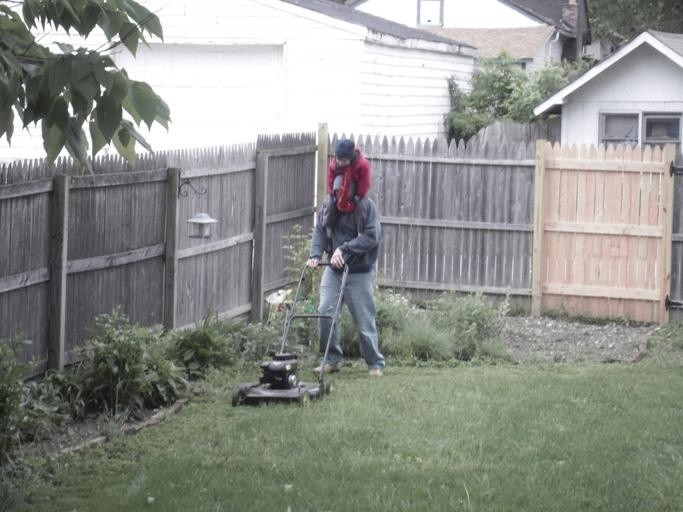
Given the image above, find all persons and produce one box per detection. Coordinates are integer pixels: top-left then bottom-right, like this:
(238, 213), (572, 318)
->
(325, 138), (370, 212)
(306, 174), (386, 378)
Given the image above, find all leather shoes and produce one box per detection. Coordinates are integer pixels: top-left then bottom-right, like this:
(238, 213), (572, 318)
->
(313, 363), (340, 374)
(369, 368), (383, 377)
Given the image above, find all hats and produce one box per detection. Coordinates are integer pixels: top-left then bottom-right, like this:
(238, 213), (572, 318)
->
(334, 139), (355, 155)
(332, 175), (356, 197)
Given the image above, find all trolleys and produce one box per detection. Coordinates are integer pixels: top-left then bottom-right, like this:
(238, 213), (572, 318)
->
(229, 260), (350, 406)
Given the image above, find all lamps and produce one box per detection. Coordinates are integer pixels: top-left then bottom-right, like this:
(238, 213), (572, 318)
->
(179, 180), (220, 241)
